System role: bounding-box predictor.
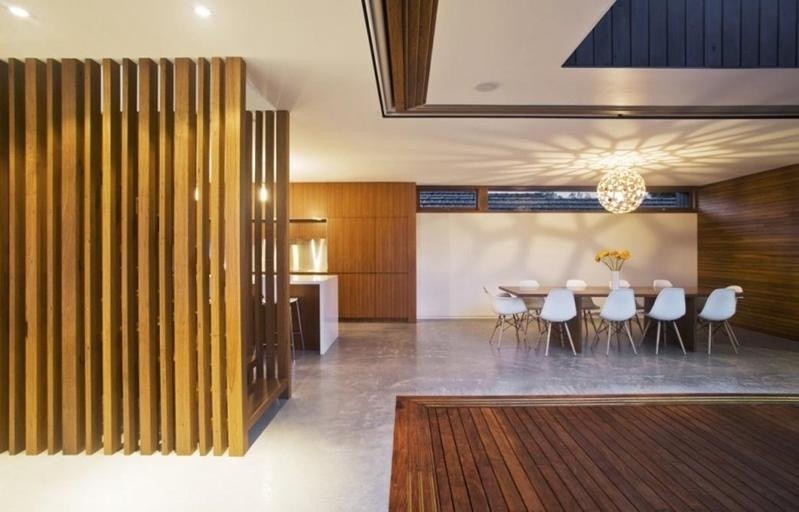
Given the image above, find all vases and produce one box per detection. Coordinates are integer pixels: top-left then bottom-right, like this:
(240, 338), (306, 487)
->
(610, 270), (620, 290)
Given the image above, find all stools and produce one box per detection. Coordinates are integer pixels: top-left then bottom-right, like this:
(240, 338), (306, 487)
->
(289, 296), (305, 359)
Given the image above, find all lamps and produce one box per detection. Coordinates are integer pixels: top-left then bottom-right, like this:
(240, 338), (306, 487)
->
(597, 166), (646, 217)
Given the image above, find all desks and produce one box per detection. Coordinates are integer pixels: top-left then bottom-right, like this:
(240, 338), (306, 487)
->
(499, 285), (744, 354)
(252, 272), (340, 356)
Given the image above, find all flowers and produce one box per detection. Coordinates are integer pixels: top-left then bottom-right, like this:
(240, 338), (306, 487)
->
(594, 248), (631, 271)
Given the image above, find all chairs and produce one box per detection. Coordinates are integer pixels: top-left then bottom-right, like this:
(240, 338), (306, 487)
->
(515, 279), (548, 336)
(653, 279), (673, 288)
(638, 288), (688, 357)
(608, 279), (642, 344)
(696, 288), (739, 355)
(566, 279), (598, 337)
(532, 288), (579, 358)
(483, 284), (531, 351)
(709, 285), (744, 347)
(589, 289), (638, 358)
